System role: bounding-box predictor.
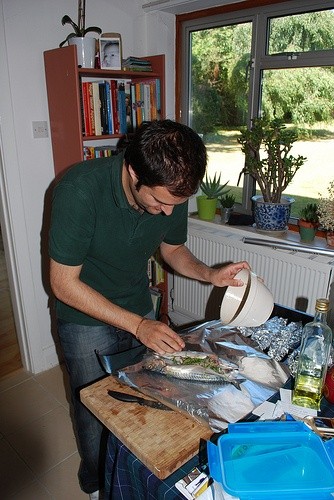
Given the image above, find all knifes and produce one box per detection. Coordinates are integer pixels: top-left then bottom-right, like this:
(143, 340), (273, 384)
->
(107, 390), (174, 411)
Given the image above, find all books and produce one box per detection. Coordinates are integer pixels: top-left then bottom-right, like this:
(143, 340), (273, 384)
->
(80, 76), (162, 137)
(83, 146), (112, 160)
(122, 55), (153, 72)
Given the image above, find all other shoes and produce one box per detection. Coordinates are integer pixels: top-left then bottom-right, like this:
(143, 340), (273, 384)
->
(89, 490), (99, 500)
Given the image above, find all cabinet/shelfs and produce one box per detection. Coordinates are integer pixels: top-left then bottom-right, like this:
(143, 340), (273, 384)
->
(43, 45), (168, 330)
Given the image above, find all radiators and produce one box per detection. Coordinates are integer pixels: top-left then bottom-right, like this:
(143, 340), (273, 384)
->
(171, 227), (334, 321)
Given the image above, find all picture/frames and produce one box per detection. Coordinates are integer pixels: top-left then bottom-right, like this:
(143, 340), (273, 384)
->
(99, 37), (122, 70)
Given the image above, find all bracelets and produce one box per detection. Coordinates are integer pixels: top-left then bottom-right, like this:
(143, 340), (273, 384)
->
(135, 317), (150, 334)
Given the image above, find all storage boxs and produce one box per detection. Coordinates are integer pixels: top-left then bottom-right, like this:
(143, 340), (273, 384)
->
(206, 420), (334, 500)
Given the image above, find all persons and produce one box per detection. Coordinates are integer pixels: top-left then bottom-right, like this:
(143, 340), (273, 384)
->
(101, 42), (119, 67)
(48, 119), (252, 500)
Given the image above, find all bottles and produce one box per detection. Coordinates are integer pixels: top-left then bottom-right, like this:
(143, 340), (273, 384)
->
(291, 298), (333, 411)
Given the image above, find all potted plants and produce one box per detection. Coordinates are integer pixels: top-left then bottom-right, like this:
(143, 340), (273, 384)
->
(196, 172), (232, 219)
(219, 194), (235, 222)
(237, 112), (307, 232)
(316, 181), (334, 246)
(59, 0), (102, 68)
(298, 203), (321, 241)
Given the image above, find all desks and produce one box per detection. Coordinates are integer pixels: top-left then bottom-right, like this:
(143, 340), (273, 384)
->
(105, 377), (334, 500)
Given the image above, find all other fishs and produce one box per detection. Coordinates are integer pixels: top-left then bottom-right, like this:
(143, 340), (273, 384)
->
(141, 359), (247, 392)
(138, 345), (240, 370)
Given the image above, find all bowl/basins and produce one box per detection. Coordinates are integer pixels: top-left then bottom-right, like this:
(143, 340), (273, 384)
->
(220, 268), (274, 327)
